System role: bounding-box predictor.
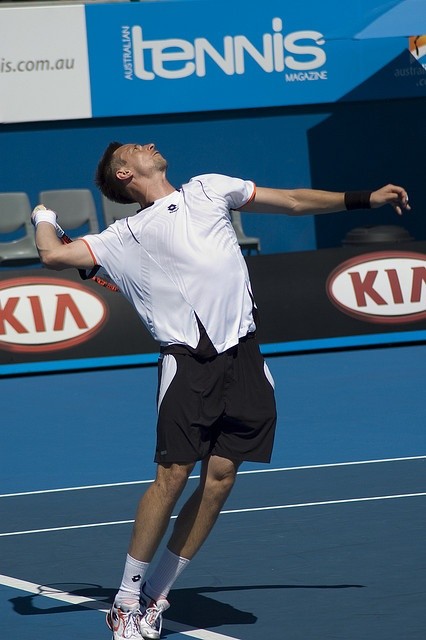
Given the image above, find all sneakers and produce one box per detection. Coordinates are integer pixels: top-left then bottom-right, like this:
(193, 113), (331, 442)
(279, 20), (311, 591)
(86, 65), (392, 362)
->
(105, 602), (144, 640)
(139, 581), (172, 639)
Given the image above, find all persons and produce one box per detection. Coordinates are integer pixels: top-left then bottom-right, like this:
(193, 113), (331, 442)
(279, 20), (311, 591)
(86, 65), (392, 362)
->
(30, 140), (411, 640)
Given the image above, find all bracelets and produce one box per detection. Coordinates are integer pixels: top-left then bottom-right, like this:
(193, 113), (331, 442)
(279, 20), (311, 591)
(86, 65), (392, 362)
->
(345, 190), (376, 212)
(33, 209), (57, 230)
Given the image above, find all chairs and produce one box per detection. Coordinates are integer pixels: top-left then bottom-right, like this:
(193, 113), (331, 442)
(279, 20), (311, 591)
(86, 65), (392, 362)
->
(38, 189), (99, 244)
(101, 191), (142, 229)
(228, 210), (261, 257)
(0, 192), (38, 262)
